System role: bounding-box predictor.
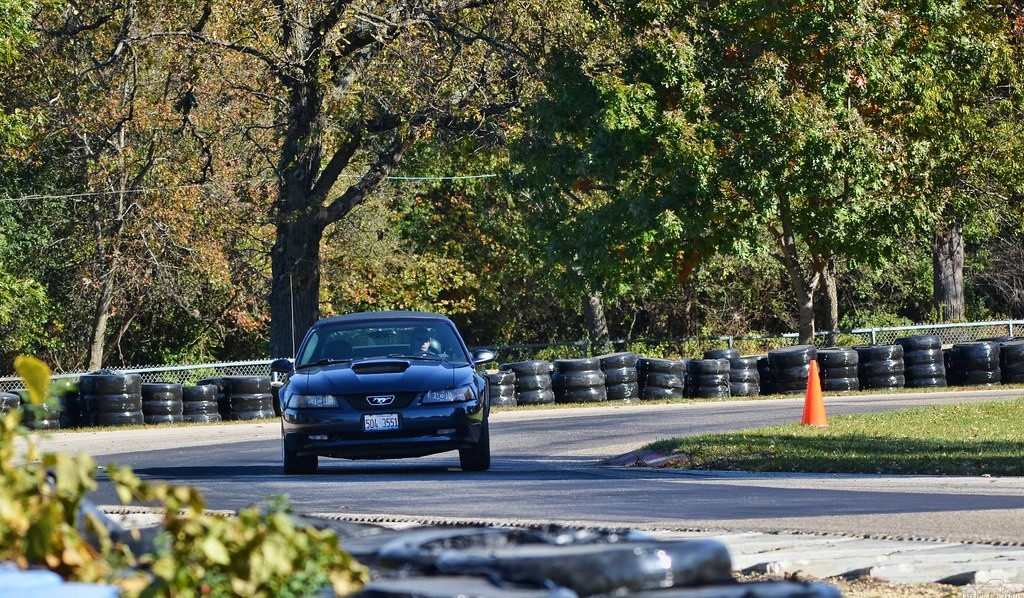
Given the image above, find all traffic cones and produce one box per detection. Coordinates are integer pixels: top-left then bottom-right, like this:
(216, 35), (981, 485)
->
(800, 359), (828, 428)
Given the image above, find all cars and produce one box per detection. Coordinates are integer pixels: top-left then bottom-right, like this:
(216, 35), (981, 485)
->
(271, 313), (495, 478)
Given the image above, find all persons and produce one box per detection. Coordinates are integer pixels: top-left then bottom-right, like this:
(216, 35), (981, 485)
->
(410, 328), (449, 358)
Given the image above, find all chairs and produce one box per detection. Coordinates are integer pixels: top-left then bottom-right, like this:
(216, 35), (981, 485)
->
(324, 340), (353, 358)
(428, 338), (441, 354)
